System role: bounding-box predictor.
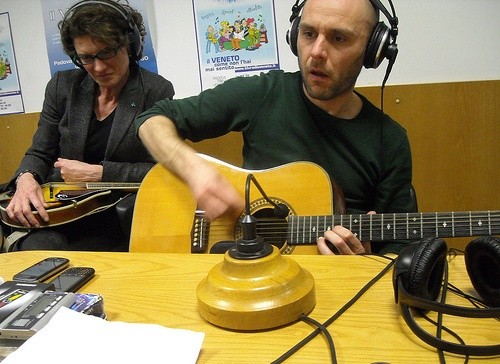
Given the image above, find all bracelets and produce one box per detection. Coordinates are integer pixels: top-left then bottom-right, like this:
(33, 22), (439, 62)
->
(14, 172), (23, 184)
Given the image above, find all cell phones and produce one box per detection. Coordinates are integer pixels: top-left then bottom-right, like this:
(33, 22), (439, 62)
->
(48, 267), (95, 294)
(13, 257), (70, 283)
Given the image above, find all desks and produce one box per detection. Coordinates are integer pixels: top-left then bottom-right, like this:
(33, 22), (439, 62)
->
(0, 251), (500, 364)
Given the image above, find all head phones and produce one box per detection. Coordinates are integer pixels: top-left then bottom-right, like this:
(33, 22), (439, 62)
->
(61, 0), (143, 56)
(393, 237), (500, 356)
(285, 0), (400, 90)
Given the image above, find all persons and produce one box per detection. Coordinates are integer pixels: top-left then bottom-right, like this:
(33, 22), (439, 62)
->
(134, 0), (419, 256)
(0, 0), (175, 253)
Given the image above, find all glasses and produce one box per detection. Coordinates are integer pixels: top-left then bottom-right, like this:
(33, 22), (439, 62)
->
(73, 44), (125, 66)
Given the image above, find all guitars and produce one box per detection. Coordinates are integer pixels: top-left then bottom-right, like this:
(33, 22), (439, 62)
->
(0, 181), (141, 229)
(127, 151), (500, 255)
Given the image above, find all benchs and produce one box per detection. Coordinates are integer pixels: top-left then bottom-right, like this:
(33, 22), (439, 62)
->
(0, 79), (500, 254)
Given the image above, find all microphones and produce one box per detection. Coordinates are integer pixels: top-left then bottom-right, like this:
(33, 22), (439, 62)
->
(195, 173), (315, 331)
